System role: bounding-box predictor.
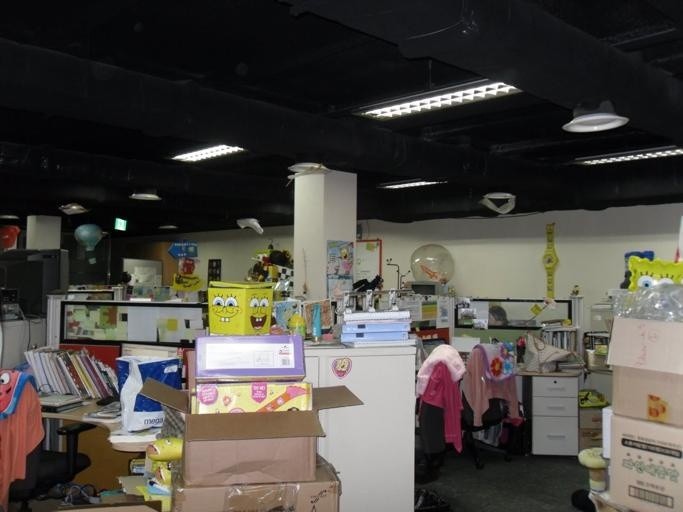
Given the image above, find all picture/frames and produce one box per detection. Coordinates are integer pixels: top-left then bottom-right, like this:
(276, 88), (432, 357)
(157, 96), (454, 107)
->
(355, 239), (382, 285)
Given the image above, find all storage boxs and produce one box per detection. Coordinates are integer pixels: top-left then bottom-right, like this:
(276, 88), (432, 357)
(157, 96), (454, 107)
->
(205, 280), (273, 334)
(170, 457), (345, 511)
(194, 335), (307, 383)
(141, 376), (362, 485)
(605, 315), (683, 512)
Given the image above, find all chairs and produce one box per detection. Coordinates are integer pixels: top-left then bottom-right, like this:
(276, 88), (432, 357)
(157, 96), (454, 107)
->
(1, 368), (97, 511)
(415, 344), (512, 511)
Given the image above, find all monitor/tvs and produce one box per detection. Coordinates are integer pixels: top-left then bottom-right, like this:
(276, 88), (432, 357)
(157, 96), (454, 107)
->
(406, 281), (441, 295)
(1, 248), (70, 316)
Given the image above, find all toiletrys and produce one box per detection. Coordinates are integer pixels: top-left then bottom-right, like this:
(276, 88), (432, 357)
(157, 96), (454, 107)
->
(311, 304), (322, 342)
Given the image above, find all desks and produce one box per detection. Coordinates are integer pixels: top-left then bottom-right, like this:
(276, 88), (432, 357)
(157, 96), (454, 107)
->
(37, 394), (161, 453)
(463, 360), (584, 458)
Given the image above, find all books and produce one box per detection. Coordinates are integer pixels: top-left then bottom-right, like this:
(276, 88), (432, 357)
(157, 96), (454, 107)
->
(24, 346), (121, 399)
(334, 310), (419, 349)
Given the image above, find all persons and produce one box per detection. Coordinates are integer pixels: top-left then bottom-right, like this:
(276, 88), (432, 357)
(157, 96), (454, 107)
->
(489, 306), (508, 325)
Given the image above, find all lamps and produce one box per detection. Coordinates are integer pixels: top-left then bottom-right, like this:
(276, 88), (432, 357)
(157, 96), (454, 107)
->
(130, 187), (161, 202)
(561, 99), (629, 137)
